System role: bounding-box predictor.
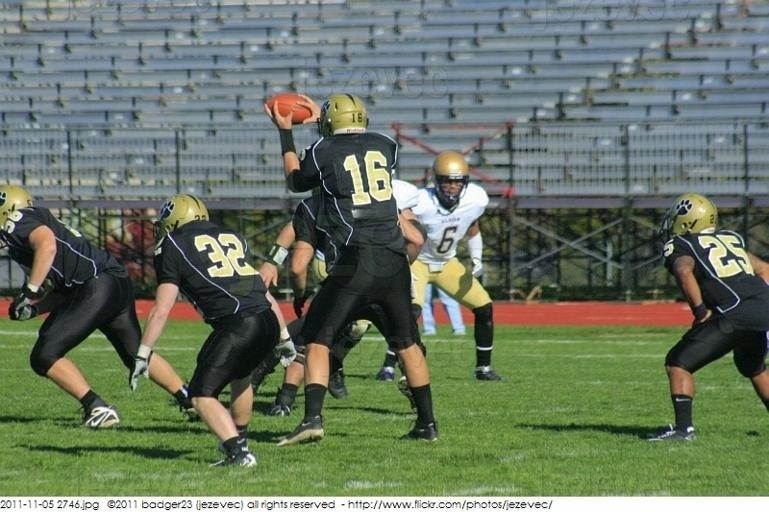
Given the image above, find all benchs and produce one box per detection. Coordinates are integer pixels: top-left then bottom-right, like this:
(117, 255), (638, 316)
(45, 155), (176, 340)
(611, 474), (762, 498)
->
(0, 1), (769, 216)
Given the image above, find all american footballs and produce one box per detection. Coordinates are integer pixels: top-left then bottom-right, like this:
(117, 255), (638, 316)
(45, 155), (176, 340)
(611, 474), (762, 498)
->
(264, 95), (313, 125)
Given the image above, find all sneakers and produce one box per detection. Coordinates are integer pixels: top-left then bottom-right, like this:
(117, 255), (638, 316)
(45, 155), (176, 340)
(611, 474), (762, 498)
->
(81, 367), (501, 468)
(649, 425), (696, 442)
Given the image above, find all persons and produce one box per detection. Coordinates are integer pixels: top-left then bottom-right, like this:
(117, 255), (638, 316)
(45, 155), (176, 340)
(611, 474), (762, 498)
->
(0, 185), (197, 427)
(256, 91), (501, 444)
(651, 191), (768, 442)
(127, 191), (282, 468)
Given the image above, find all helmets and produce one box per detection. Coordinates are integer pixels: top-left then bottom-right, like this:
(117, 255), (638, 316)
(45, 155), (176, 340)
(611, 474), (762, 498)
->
(317, 94), (369, 136)
(154, 194), (208, 241)
(431, 151), (468, 206)
(660, 195), (717, 241)
(0, 186), (32, 230)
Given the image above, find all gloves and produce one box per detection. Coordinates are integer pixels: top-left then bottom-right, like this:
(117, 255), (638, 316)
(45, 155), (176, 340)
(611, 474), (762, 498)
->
(130, 357), (151, 390)
(275, 338), (298, 369)
(470, 259), (484, 278)
(9, 285), (42, 320)
